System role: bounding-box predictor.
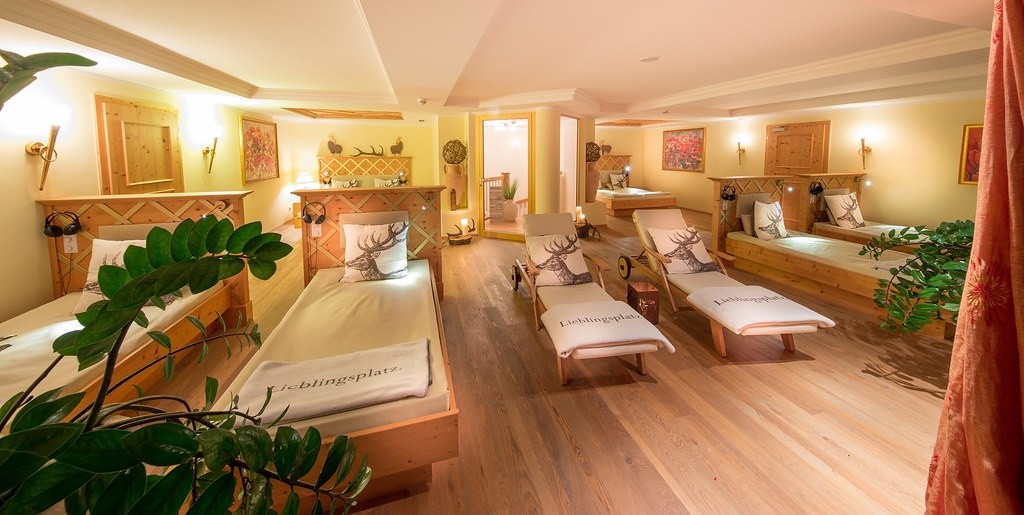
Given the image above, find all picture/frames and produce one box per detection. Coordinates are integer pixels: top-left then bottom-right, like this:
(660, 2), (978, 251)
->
(661, 127), (707, 172)
(958, 122), (985, 185)
(238, 114), (280, 185)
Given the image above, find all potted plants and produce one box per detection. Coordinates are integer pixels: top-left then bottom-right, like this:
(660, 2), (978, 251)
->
(859, 222), (975, 341)
(499, 175), (520, 223)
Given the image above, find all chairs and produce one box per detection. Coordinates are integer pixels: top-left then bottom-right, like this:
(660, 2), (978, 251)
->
(510, 211), (677, 387)
(617, 208), (835, 359)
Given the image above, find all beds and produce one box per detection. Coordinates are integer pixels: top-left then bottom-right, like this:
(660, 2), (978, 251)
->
(792, 171), (967, 254)
(598, 153), (676, 216)
(707, 175), (937, 321)
(322, 174), (399, 189)
(0, 189), (258, 438)
(163, 186), (461, 515)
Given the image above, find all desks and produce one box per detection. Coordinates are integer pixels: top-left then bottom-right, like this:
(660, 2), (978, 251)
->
(627, 282), (659, 324)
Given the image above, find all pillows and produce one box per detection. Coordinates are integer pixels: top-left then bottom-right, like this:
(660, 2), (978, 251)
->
(605, 182), (614, 190)
(740, 215), (754, 235)
(646, 224), (717, 275)
(330, 179), (358, 188)
(824, 192), (866, 229)
(338, 220), (409, 283)
(826, 209), (838, 226)
(754, 200), (789, 239)
(527, 233), (592, 288)
(374, 178), (398, 188)
(69, 238), (176, 315)
(610, 174), (626, 189)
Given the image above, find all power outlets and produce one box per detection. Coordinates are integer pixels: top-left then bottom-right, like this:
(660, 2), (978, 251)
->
(723, 200), (728, 210)
(313, 221), (322, 238)
(810, 194), (814, 202)
(64, 234), (78, 254)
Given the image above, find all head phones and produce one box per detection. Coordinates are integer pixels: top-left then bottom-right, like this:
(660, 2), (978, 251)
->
(809, 182), (823, 195)
(43, 212), (81, 238)
(302, 202), (326, 224)
(722, 184), (736, 201)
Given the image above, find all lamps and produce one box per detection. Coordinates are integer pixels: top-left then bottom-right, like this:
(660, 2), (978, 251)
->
(737, 140), (746, 165)
(202, 135), (219, 173)
(461, 218), (469, 234)
(857, 135), (873, 170)
(576, 206), (586, 224)
(26, 121), (63, 191)
(297, 173), (314, 188)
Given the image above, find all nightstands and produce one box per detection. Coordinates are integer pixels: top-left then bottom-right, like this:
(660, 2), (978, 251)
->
(293, 203), (301, 229)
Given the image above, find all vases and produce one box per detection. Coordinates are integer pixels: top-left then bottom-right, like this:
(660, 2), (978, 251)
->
(586, 161), (599, 204)
(443, 163), (464, 210)
